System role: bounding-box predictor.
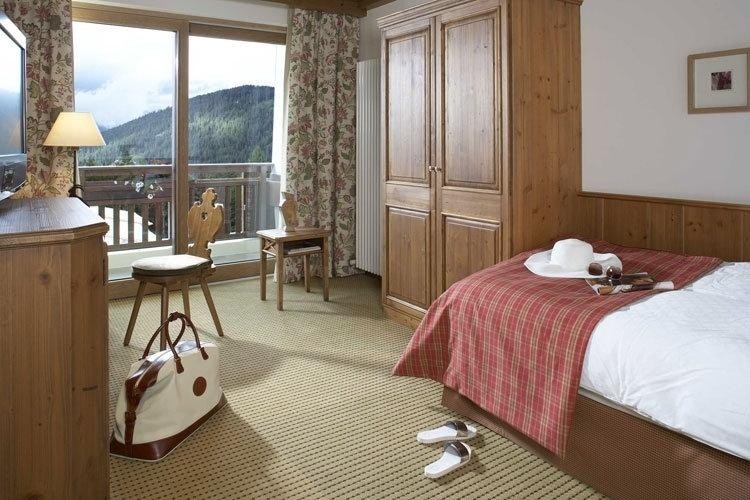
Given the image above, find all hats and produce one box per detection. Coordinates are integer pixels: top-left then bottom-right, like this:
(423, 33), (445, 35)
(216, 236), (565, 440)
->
(523, 238), (623, 279)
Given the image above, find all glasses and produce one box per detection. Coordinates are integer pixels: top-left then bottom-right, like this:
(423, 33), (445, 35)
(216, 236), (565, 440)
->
(588, 262), (624, 281)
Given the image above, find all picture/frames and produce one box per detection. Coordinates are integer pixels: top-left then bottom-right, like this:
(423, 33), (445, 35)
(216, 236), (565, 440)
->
(685, 48), (750, 115)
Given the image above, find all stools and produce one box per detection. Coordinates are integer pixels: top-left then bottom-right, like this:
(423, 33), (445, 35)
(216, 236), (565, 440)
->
(255, 225), (332, 311)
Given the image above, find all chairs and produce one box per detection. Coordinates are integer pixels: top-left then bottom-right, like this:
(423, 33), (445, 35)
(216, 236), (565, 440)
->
(123, 184), (224, 352)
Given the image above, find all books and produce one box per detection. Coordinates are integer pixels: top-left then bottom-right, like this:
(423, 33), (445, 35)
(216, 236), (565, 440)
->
(284, 243), (321, 255)
(585, 272), (674, 295)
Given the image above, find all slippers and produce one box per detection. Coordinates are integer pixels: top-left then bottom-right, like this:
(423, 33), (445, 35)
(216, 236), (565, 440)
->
(416, 419), (478, 444)
(424, 441), (472, 479)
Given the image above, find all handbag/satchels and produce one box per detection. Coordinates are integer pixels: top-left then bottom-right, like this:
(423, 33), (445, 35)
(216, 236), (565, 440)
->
(109, 310), (228, 464)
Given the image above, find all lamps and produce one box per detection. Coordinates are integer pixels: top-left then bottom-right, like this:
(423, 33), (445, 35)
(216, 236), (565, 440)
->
(41, 112), (107, 207)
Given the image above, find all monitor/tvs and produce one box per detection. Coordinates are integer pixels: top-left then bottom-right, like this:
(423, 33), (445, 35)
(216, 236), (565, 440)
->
(0, 9), (28, 203)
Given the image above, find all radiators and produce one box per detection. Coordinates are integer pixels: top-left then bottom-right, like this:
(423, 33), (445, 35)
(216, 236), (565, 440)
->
(355, 56), (382, 278)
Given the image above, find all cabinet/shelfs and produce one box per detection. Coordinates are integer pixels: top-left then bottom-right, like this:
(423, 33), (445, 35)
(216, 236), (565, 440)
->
(375, 2), (583, 332)
(1, 196), (112, 500)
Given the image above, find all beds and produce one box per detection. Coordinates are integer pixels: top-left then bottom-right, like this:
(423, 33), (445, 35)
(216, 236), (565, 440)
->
(388, 237), (750, 499)
